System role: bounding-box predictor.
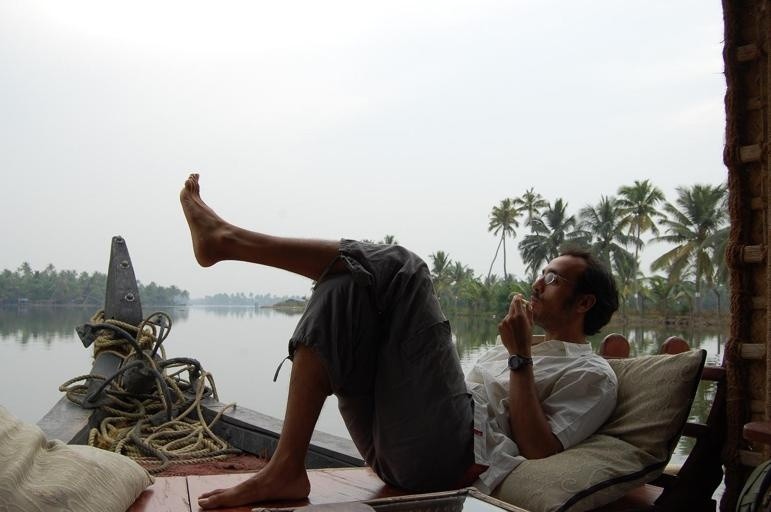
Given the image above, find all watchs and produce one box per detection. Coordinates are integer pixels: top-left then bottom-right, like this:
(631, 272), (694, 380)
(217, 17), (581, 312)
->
(507, 355), (532, 372)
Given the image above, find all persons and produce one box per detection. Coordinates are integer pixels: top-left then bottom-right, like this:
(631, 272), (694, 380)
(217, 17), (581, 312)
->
(181, 174), (619, 509)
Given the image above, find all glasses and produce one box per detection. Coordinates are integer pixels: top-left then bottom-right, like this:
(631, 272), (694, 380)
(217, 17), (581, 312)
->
(535, 270), (580, 287)
